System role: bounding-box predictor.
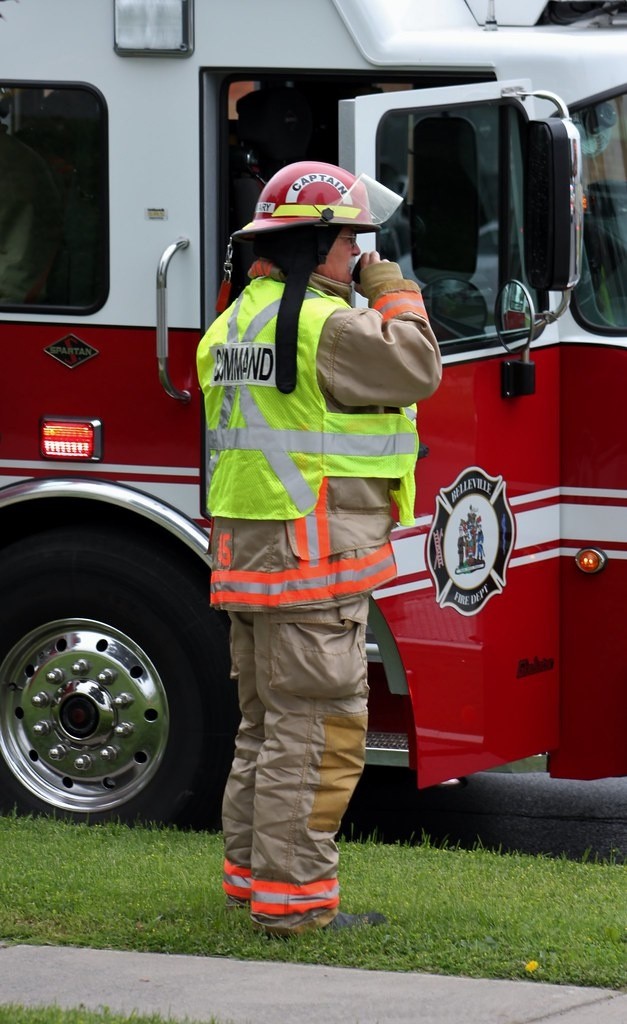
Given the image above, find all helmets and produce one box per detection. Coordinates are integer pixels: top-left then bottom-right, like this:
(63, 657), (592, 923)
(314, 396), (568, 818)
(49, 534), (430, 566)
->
(229, 161), (404, 245)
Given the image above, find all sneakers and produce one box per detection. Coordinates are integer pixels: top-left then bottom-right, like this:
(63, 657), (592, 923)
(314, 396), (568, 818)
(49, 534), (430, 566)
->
(226, 895), (251, 912)
(256, 911), (388, 943)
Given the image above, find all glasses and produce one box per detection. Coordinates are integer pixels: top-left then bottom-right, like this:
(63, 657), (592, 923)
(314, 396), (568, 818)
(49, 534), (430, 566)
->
(334, 231), (358, 248)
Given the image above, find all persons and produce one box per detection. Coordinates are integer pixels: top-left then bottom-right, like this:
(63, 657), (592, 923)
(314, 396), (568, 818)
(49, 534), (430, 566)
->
(1, 124), (98, 311)
(195, 162), (443, 939)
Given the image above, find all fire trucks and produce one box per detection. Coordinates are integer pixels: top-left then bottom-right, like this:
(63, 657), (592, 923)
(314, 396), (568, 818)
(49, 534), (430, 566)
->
(2, 0), (627, 821)
